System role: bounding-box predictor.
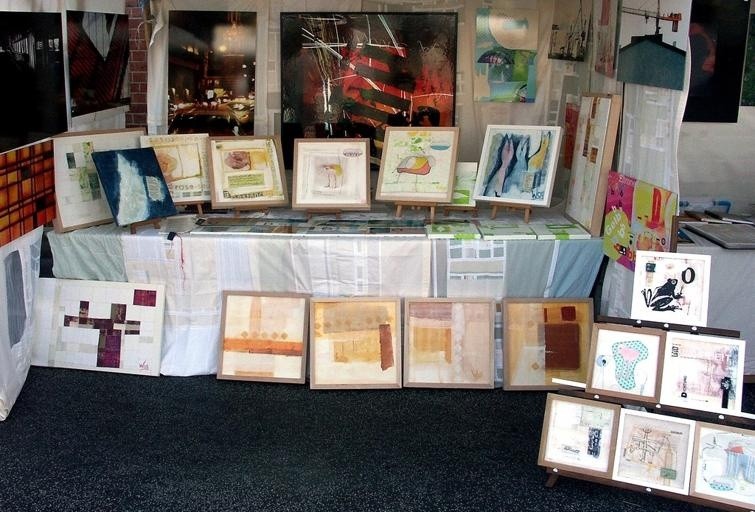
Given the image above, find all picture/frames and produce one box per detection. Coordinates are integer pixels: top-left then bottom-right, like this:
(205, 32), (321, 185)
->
(629, 251), (715, 325)
(688, 421), (754, 511)
(471, 121), (565, 223)
(500, 294), (598, 394)
(613, 408), (697, 500)
(582, 320), (667, 408)
(537, 391), (622, 483)
(374, 122), (462, 219)
(307, 293), (404, 393)
(401, 295), (497, 392)
(90, 146), (176, 236)
(218, 286), (309, 388)
(659, 331), (748, 418)
(204, 133), (289, 214)
(443, 159), (479, 218)
(137, 130), (212, 216)
(560, 94), (625, 238)
(47, 125), (146, 234)
(291, 135), (374, 216)
